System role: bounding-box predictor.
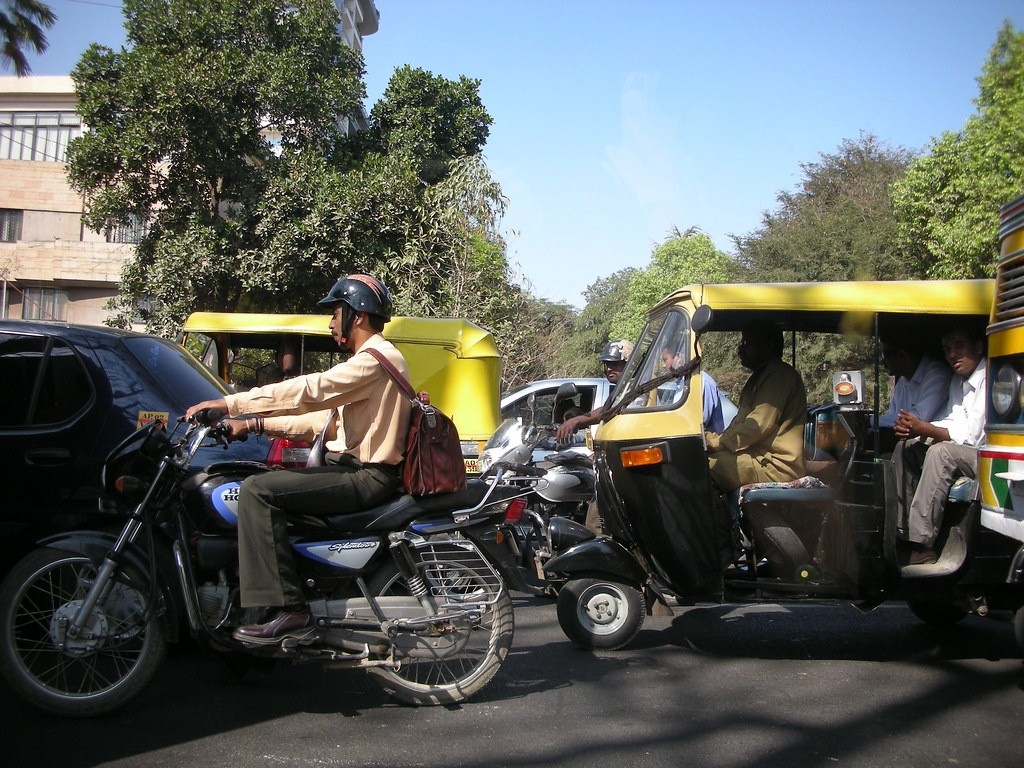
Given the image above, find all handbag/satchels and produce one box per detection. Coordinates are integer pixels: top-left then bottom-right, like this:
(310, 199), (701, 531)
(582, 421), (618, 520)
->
(401, 391), (465, 497)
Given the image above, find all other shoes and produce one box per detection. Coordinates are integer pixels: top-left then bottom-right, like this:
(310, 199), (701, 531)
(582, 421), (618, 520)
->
(728, 561), (781, 582)
(908, 548), (939, 566)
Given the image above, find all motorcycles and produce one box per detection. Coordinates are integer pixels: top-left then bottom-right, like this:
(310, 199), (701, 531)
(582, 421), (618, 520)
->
(1, 401), (551, 721)
(982, 193), (1024, 659)
(541, 278), (999, 653)
(174, 311), (504, 480)
(468, 381), (597, 598)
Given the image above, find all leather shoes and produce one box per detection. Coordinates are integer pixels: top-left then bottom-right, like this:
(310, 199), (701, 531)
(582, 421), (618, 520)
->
(233, 607), (317, 644)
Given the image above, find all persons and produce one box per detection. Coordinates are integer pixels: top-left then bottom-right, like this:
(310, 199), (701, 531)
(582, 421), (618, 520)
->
(557, 340), (725, 446)
(186, 274), (414, 647)
(705, 326), (806, 573)
(864, 331), (988, 564)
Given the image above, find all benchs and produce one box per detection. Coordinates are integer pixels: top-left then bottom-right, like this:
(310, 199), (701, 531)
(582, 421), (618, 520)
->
(747, 402), (845, 506)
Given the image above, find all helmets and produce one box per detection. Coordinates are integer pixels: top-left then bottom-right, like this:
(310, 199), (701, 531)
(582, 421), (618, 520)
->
(598, 339), (636, 362)
(316, 274), (392, 322)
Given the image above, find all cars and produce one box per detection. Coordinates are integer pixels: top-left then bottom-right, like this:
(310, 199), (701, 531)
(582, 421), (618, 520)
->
(500, 377), (739, 490)
(0, 316), (316, 658)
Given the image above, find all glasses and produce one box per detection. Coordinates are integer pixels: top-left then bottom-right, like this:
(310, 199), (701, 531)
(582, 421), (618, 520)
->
(737, 339), (749, 348)
